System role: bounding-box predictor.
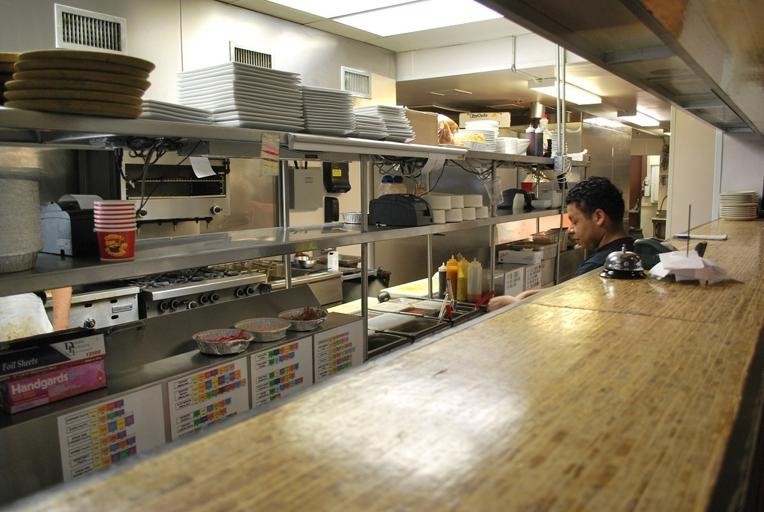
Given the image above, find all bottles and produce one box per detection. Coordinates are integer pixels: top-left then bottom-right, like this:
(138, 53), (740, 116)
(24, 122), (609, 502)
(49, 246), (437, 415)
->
(393, 175), (406, 194)
(378, 175), (395, 196)
(525, 113), (552, 157)
(436, 254), (483, 302)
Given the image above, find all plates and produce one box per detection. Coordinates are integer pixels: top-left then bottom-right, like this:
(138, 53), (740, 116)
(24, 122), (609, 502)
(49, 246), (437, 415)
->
(529, 182), (577, 210)
(352, 104), (416, 144)
(718, 190), (757, 221)
(190, 306), (327, 355)
(177, 61), (305, 133)
(300, 86), (354, 137)
(440, 119), (499, 152)
(0, 177), (43, 273)
(139, 96), (211, 125)
(2, 48), (156, 120)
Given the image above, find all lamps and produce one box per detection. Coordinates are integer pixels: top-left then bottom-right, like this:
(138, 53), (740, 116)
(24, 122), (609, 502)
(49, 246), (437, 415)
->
(617, 109), (661, 128)
(526, 79), (602, 106)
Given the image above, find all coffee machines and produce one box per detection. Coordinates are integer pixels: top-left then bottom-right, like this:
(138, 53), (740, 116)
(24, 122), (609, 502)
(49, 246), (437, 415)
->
(64, 150), (231, 240)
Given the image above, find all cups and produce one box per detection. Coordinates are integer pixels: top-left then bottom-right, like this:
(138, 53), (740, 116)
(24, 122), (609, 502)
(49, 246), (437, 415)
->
(482, 178), (504, 206)
(512, 193), (525, 209)
(425, 192), (490, 223)
(90, 198), (138, 262)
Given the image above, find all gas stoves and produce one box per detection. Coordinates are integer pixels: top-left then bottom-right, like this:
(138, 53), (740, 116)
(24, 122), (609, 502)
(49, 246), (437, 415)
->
(136, 264), (268, 314)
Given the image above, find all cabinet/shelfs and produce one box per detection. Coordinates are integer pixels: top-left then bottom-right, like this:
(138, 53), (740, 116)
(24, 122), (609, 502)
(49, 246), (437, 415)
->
(0, 107), (592, 351)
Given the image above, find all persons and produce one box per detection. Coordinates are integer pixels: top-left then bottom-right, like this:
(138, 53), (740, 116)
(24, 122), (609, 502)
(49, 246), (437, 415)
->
(487, 176), (637, 313)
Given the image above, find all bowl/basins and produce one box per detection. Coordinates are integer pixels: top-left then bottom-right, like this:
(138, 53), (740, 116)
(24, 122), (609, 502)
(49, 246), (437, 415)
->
(299, 259), (316, 268)
(496, 136), (529, 156)
(341, 210), (371, 224)
